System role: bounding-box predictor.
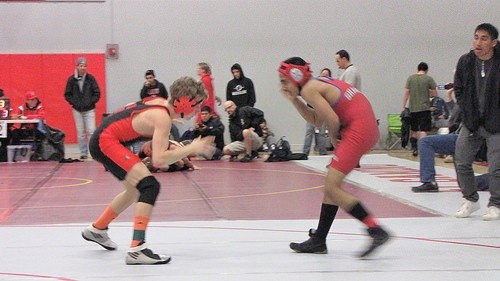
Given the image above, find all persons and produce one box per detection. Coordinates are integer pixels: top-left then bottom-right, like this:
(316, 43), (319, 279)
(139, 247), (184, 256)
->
(276, 54), (391, 261)
(222, 62), (276, 158)
(10, 89), (47, 160)
(64, 58), (101, 160)
(80, 75), (222, 266)
(409, 77), (495, 195)
(219, 100), (268, 162)
(324, 50), (363, 168)
(117, 63), (225, 173)
(403, 60), (489, 165)
(300, 68), (334, 156)
(0, 88), (13, 162)
(451, 22), (500, 224)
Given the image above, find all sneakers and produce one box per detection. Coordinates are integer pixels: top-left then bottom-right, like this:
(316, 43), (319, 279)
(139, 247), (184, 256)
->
(289, 229), (328, 253)
(360, 226), (389, 258)
(455, 199), (480, 218)
(82, 223), (117, 250)
(240, 153), (256, 161)
(482, 205), (500, 220)
(125, 241), (171, 264)
(250, 150), (258, 157)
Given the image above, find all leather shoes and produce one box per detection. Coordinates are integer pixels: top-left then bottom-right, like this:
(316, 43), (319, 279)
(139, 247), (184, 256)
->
(412, 182), (439, 192)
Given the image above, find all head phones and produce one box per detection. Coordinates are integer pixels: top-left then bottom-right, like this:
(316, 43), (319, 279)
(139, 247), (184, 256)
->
(278, 61), (309, 85)
(172, 95), (204, 118)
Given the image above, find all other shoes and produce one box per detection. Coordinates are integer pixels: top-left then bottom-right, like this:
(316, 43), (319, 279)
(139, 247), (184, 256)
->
(270, 144), (276, 150)
(262, 144), (268, 151)
(320, 149), (327, 155)
(80, 155), (87, 159)
(355, 162), (360, 168)
(412, 149), (418, 155)
(444, 155), (454, 163)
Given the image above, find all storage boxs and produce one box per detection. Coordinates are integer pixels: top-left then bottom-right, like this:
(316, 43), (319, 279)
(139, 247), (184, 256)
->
(5, 144), (33, 162)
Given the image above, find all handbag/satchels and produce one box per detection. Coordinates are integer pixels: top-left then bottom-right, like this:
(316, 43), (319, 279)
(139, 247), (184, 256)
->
(37, 120), (66, 160)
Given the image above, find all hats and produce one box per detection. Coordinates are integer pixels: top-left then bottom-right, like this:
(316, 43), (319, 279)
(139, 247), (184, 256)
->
(76, 58), (86, 65)
(25, 91), (37, 100)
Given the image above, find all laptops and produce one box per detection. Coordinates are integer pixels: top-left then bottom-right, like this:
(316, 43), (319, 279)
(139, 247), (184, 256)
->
(0, 98), (11, 120)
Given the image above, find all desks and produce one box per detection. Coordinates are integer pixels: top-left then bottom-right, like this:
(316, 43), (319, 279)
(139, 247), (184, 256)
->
(0, 119), (47, 161)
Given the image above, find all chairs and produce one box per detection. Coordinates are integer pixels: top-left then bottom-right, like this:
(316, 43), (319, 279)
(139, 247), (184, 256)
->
(387, 115), (402, 151)
